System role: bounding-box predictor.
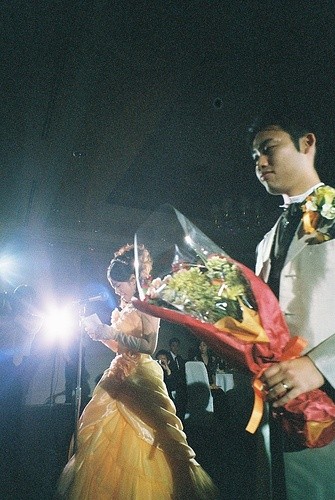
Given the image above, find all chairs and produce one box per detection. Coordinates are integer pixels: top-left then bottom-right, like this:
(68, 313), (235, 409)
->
(184, 361), (215, 421)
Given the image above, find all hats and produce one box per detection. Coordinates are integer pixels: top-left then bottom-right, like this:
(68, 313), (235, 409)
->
(237, 113), (319, 150)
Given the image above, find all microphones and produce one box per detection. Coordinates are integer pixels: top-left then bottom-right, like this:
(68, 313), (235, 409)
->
(77, 293), (108, 304)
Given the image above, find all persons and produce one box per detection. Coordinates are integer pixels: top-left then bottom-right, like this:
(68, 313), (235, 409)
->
(154, 337), (225, 396)
(0, 285), (47, 404)
(53, 243), (219, 500)
(247, 114), (335, 500)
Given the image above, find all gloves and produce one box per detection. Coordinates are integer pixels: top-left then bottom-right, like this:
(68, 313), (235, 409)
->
(85, 322), (142, 354)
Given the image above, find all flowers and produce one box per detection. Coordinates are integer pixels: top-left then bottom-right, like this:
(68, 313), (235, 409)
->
(302, 184), (335, 233)
(111, 308), (120, 325)
(138, 253), (257, 323)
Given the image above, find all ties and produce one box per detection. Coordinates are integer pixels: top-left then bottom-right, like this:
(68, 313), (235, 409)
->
(273, 203), (302, 260)
(175, 357), (179, 369)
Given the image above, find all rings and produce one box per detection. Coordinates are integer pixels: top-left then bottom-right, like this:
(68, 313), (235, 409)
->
(282, 381), (289, 392)
